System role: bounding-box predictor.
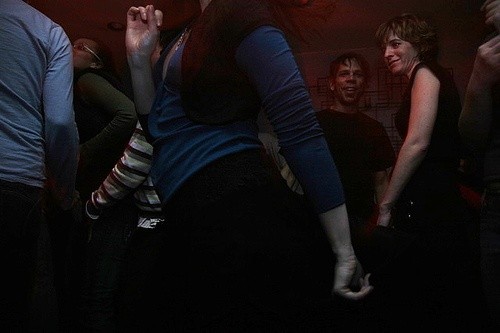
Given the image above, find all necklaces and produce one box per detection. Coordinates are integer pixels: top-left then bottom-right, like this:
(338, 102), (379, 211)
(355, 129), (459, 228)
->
(176, 27), (187, 52)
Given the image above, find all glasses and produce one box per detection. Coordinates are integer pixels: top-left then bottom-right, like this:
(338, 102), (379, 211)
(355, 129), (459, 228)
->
(72, 43), (104, 67)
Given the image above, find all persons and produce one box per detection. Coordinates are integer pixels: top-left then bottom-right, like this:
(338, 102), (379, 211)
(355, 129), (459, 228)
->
(125, 0), (374, 332)
(64, 35), (138, 193)
(0, 0), (78, 333)
(457, 0), (499, 268)
(312, 52), (399, 250)
(374, 12), (469, 229)
(79, 28), (306, 333)
